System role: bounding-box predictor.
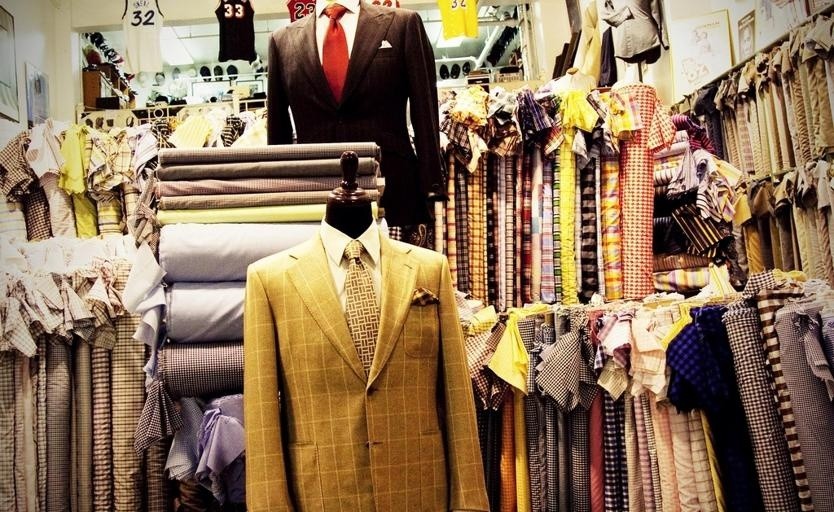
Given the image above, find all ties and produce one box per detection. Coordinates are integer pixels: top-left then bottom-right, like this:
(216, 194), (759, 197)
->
(322, 6), (350, 102)
(343, 240), (380, 377)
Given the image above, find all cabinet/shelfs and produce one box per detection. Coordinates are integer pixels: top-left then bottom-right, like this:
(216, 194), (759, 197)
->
(670, 2), (834, 192)
(83, 32), (138, 103)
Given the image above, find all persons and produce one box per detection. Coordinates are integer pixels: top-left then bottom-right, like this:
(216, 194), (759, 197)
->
(243, 188), (492, 512)
(268, 0), (449, 246)
